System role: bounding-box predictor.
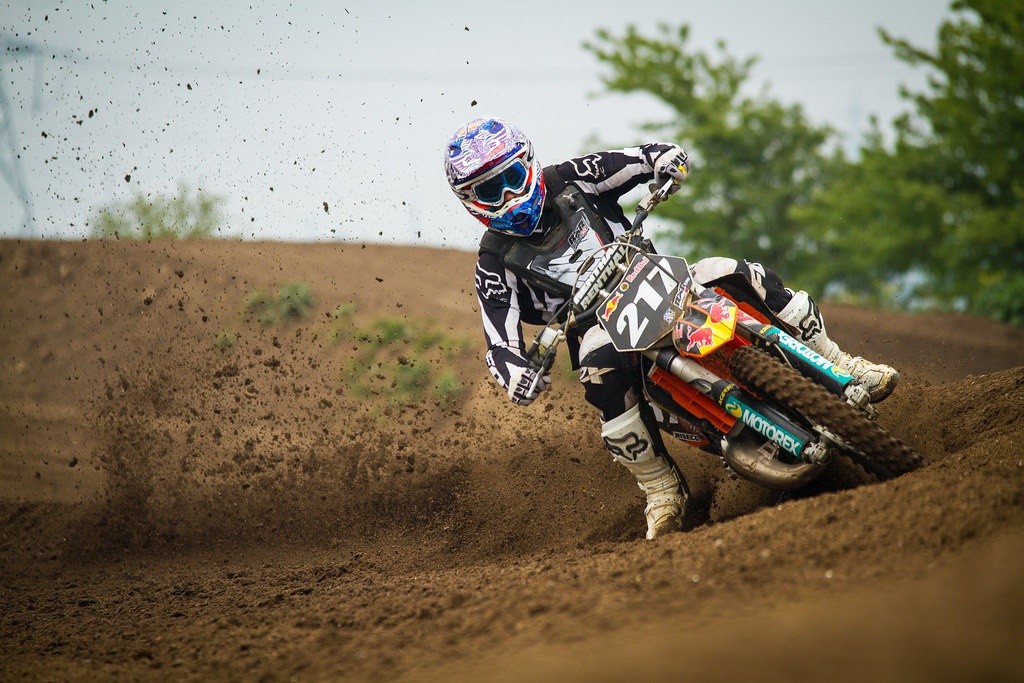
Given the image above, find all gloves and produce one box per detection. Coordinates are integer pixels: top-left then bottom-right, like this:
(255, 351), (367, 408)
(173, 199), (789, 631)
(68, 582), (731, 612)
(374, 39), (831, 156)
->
(640, 141), (690, 195)
(484, 340), (552, 406)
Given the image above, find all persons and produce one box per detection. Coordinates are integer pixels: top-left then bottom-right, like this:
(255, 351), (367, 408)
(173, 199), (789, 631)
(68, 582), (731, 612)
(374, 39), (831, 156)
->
(444, 117), (900, 540)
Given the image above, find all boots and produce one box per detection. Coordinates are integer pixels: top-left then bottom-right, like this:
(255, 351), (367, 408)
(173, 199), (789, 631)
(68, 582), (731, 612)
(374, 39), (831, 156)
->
(775, 285), (900, 405)
(599, 401), (687, 542)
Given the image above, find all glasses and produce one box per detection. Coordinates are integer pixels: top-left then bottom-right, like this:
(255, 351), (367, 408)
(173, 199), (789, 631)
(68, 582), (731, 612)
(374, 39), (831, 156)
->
(471, 158), (530, 207)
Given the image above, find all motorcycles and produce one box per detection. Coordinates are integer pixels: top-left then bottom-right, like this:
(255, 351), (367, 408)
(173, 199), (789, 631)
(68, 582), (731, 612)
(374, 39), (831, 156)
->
(523, 176), (930, 508)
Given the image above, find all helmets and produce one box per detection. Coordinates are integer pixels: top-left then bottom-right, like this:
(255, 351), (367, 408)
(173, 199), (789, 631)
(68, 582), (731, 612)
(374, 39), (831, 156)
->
(444, 118), (547, 237)
